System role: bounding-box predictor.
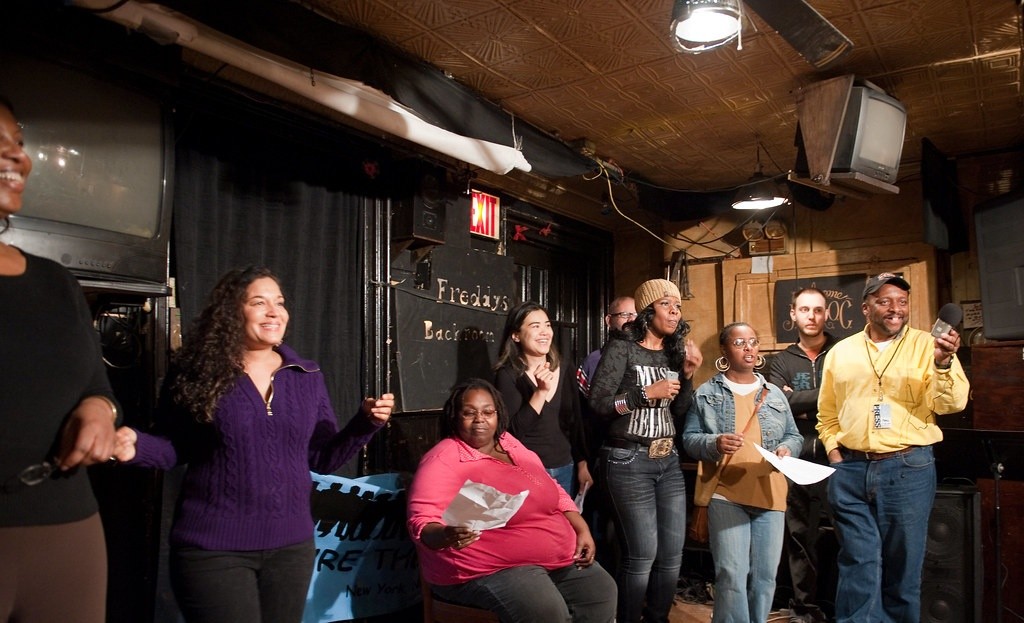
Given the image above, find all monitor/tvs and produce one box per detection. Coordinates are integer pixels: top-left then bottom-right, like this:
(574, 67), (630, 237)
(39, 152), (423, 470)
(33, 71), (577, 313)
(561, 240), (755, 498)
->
(0, 27), (177, 282)
(794, 87), (907, 184)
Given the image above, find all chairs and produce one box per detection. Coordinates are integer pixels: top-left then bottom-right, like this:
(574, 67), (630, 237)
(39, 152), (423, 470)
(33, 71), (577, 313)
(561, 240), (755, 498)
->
(402, 471), (502, 623)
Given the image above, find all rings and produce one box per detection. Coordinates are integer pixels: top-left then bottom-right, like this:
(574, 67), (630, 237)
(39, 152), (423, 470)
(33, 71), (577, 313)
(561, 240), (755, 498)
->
(457, 540), (461, 545)
(591, 555), (594, 558)
(110, 456), (115, 460)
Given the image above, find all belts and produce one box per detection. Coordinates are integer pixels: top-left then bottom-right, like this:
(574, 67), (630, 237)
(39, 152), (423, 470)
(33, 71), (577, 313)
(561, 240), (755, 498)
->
(842, 448), (913, 461)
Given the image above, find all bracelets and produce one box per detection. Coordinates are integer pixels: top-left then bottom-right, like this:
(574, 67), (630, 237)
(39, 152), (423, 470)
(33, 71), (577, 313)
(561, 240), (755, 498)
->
(614, 393), (635, 415)
(97, 395), (117, 419)
(641, 386), (651, 403)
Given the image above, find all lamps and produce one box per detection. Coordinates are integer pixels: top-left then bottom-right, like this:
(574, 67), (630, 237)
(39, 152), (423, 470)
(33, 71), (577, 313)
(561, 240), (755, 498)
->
(731, 134), (789, 211)
(742, 219), (788, 256)
(668, 0), (748, 54)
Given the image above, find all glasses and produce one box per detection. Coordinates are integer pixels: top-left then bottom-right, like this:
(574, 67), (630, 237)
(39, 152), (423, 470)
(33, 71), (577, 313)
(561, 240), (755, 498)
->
(610, 311), (638, 319)
(459, 408), (497, 419)
(19, 457), (62, 486)
(724, 338), (760, 349)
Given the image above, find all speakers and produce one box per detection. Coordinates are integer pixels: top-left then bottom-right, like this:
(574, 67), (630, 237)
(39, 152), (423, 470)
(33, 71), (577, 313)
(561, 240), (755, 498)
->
(973, 187), (1023, 339)
(919, 484), (985, 623)
(391, 158), (448, 244)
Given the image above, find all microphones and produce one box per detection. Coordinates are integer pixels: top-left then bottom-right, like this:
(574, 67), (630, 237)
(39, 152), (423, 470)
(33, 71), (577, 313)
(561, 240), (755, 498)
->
(931, 303), (963, 338)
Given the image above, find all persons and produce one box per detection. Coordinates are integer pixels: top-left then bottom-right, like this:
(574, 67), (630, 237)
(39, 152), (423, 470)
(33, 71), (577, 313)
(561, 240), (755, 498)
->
(769, 272), (970, 623)
(406, 377), (618, 623)
(0, 95), (394, 623)
(682, 322), (804, 623)
(574, 278), (703, 623)
(487, 300), (594, 501)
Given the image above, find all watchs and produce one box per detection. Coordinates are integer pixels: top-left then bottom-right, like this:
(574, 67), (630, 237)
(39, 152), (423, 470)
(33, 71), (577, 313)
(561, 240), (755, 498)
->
(934, 354), (954, 369)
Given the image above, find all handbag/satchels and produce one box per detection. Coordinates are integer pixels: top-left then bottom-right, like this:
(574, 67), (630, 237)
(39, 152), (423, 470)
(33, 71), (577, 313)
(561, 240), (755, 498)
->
(689, 506), (708, 543)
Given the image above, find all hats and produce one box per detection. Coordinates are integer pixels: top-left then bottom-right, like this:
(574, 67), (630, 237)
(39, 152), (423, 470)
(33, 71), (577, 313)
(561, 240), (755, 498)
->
(635, 279), (681, 315)
(863, 273), (911, 299)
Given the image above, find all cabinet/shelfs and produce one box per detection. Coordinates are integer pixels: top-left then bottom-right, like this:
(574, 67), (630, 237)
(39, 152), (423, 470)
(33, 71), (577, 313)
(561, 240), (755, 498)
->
(75, 277), (173, 623)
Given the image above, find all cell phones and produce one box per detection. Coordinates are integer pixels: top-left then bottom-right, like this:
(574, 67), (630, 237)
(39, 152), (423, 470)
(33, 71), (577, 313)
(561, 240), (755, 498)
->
(665, 371), (678, 380)
